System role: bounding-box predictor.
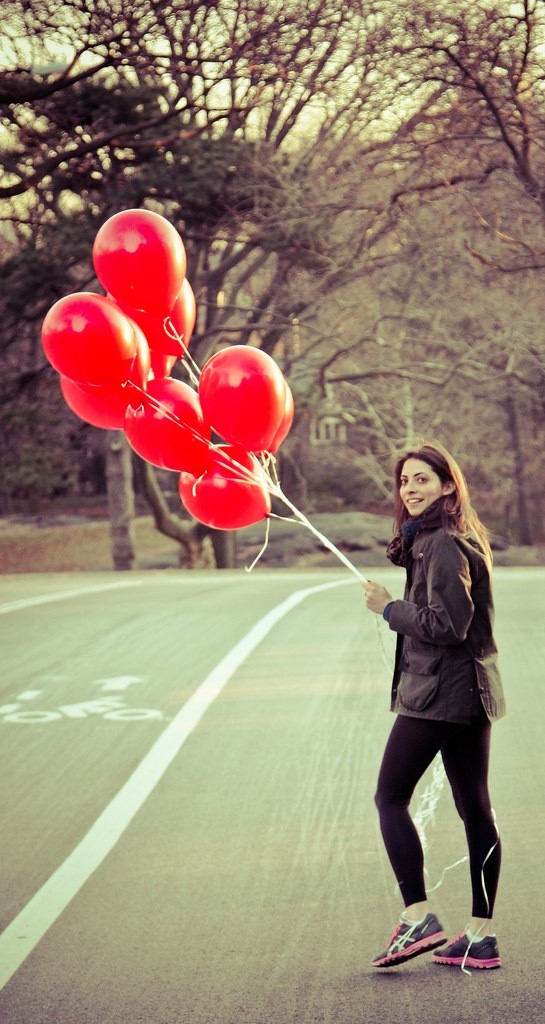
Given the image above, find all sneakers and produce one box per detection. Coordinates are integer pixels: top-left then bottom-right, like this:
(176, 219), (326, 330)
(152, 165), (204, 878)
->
(432, 934), (501, 968)
(371, 913), (447, 967)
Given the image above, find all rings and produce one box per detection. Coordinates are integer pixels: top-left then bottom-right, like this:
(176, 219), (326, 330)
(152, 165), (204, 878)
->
(364, 592), (366, 595)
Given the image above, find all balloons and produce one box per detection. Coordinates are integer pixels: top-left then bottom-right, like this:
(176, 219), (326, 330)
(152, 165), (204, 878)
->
(40, 209), (294, 532)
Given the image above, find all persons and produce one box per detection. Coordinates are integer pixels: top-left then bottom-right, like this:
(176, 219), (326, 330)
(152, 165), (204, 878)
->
(361, 445), (507, 970)
